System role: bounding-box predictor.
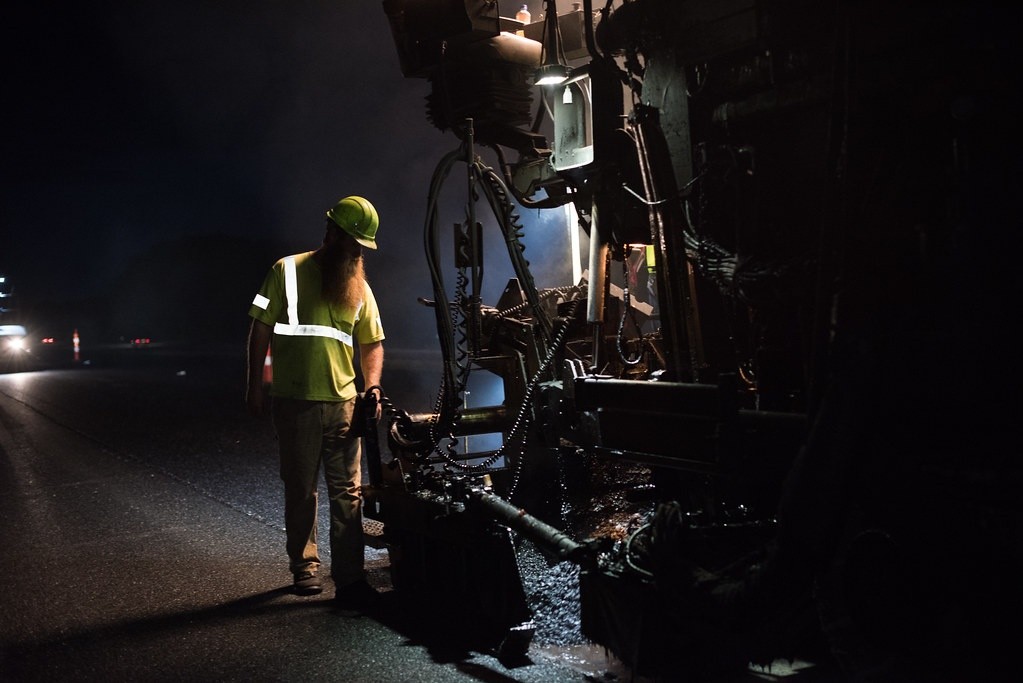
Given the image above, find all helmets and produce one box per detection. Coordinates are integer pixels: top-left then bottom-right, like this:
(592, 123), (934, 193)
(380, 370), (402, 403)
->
(327, 196), (379, 250)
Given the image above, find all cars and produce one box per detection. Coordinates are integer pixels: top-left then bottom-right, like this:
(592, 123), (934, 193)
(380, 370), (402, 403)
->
(0, 326), (32, 367)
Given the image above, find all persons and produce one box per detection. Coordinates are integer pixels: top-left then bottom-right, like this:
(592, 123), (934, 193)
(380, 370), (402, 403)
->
(245, 196), (385, 603)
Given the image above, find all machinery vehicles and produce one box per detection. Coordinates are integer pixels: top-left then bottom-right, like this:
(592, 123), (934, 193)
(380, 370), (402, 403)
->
(343, 0), (1023, 682)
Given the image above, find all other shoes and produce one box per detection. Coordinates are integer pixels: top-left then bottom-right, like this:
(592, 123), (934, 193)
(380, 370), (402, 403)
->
(335, 578), (387, 612)
(293, 566), (322, 593)
